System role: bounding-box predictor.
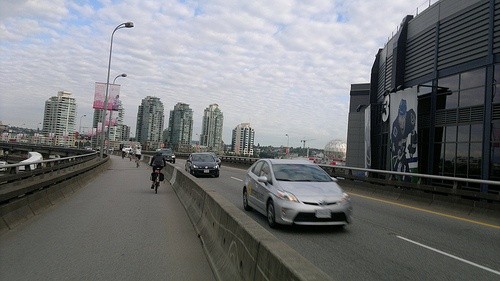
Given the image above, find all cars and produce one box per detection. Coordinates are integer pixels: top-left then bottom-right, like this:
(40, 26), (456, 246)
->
(242, 159), (354, 231)
(158, 148), (176, 164)
(185, 152), (220, 177)
(0, 161), (8, 172)
(204, 152), (222, 168)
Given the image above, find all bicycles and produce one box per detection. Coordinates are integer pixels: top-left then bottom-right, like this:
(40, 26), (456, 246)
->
(151, 167), (164, 194)
(135, 156), (141, 168)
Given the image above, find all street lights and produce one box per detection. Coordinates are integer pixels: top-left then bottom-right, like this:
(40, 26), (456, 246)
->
(106, 73), (127, 156)
(78, 115), (87, 150)
(99, 21), (134, 158)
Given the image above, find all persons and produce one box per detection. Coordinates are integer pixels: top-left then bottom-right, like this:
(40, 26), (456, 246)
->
(150, 149), (166, 189)
(391, 100), (417, 183)
(135, 146), (142, 166)
(122, 146), (132, 157)
